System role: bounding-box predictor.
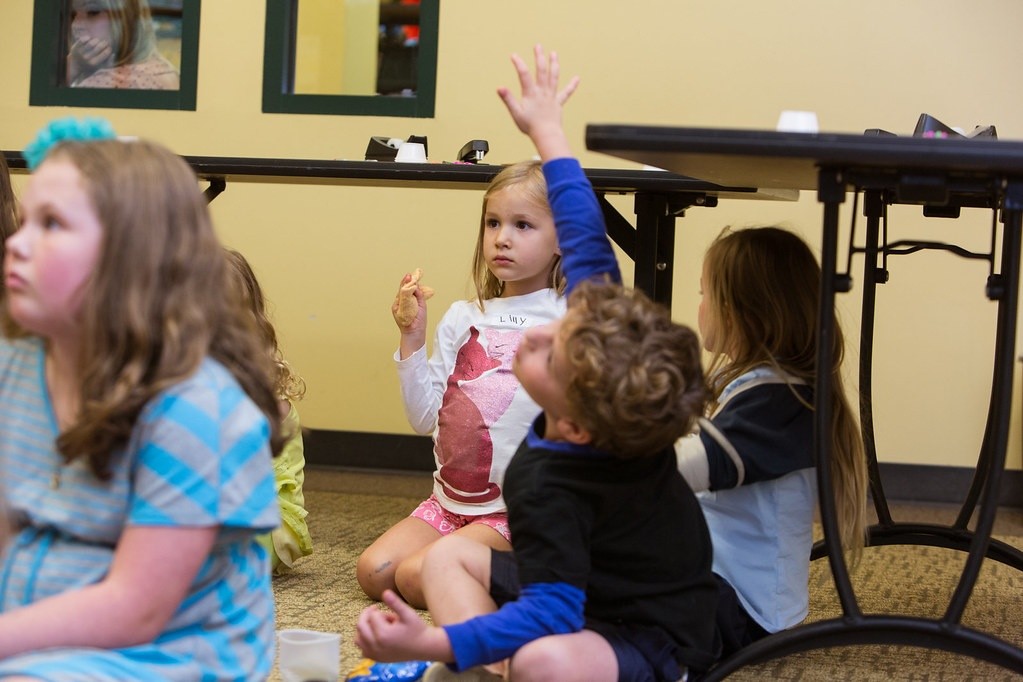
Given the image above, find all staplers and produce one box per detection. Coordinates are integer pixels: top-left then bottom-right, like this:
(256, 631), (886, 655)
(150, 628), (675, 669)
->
(456, 139), (490, 165)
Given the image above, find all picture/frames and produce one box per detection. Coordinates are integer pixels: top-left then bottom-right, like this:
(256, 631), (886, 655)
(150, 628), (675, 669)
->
(27, 0), (202, 114)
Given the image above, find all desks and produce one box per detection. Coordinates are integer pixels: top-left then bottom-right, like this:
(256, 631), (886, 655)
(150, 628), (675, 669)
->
(584, 123), (1023, 682)
(0, 151), (799, 321)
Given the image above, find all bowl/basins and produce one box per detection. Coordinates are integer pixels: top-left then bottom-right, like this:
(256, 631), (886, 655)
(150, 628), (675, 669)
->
(775, 111), (819, 134)
(394, 142), (428, 163)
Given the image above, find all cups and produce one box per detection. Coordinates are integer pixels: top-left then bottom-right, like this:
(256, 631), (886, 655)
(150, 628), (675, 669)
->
(279, 630), (341, 682)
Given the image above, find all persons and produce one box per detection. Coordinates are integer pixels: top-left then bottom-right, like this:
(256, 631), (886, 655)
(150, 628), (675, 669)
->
(216, 247), (311, 576)
(0, 141), (272, 682)
(354, 41), (720, 682)
(65, 0), (180, 89)
(357, 160), (569, 612)
(674, 230), (866, 650)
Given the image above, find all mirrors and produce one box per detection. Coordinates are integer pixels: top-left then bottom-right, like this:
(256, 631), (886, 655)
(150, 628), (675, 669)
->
(260, 0), (441, 119)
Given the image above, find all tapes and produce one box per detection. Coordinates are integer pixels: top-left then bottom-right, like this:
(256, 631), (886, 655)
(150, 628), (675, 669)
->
(387, 137), (404, 149)
